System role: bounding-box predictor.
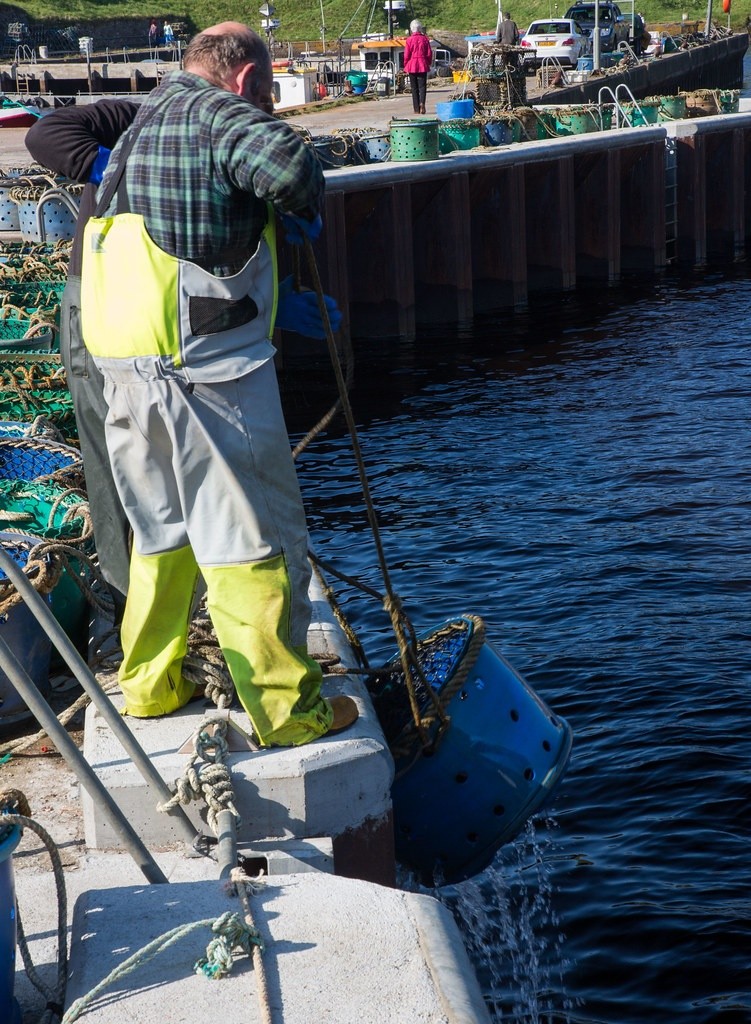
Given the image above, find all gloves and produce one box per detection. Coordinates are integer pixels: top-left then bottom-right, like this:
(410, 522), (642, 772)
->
(281, 212), (321, 247)
(88, 146), (111, 183)
(274, 276), (342, 342)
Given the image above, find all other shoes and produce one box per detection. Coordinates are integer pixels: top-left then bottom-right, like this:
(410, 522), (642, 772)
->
(420, 103), (425, 114)
(185, 680), (208, 704)
(317, 695), (359, 738)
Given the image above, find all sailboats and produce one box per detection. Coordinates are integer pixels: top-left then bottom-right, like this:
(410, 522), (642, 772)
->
(317, 0), (451, 98)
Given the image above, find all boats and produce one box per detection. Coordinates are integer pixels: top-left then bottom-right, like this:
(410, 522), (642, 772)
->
(0, 106), (40, 126)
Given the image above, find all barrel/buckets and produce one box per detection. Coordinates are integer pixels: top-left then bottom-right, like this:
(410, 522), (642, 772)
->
(434, 98), (474, 121)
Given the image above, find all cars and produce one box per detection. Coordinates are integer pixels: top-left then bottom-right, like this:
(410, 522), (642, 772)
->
(617, 14), (644, 55)
(520, 19), (591, 69)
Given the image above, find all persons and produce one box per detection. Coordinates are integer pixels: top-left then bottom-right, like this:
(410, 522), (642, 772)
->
(163, 20), (174, 47)
(497, 12), (519, 65)
(403, 20), (433, 114)
(24, 99), (141, 646)
(638, 12), (645, 28)
(149, 19), (158, 48)
(82, 21), (360, 748)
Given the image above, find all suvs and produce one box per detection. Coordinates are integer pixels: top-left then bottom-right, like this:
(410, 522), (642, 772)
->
(564, 0), (621, 52)
